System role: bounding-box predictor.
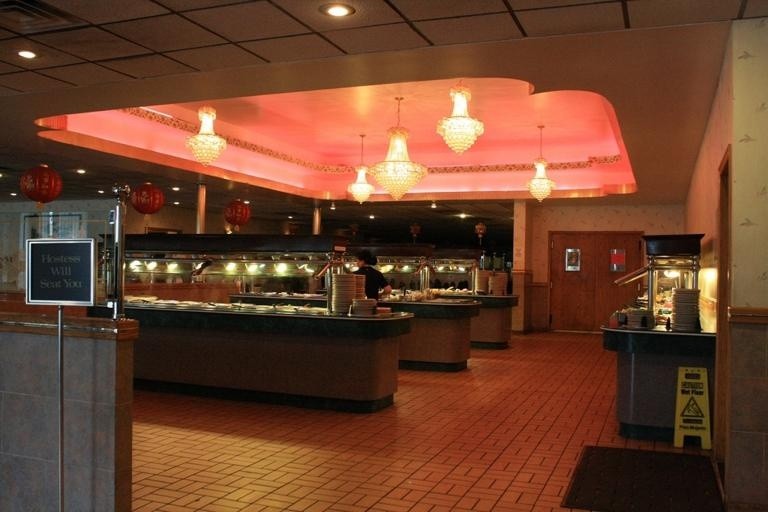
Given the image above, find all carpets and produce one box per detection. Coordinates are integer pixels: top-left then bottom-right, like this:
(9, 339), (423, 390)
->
(560, 446), (722, 512)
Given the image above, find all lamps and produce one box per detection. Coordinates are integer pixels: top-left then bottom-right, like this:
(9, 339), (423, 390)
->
(186, 105), (227, 165)
(348, 135), (374, 205)
(528, 126), (556, 202)
(435, 84), (484, 153)
(368, 98), (427, 200)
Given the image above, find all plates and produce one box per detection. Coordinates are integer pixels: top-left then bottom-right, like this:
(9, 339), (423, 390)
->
(630, 309), (654, 330)
(474, 269), (508, 296)
(670, 287), (701, 333)
(330, 272), (379, 316)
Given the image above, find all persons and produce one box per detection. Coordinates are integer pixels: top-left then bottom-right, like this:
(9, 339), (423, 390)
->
(351, 247), (391, 309)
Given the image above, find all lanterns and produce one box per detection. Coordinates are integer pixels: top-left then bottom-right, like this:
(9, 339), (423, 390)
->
(348, 221), (359, 237)
(408, 221), (421, 245)
(127, 177), (166, 223)
(223, 195), (252, 234)
(17, 161), (63, 212)
(474, 222), (487, 247)
